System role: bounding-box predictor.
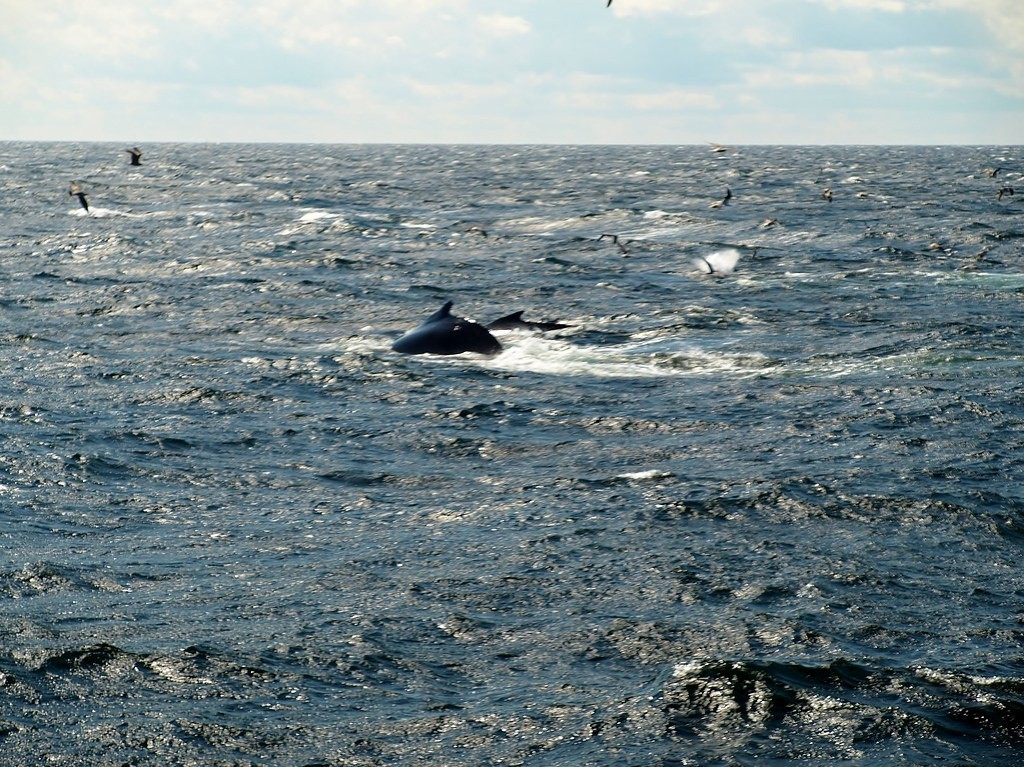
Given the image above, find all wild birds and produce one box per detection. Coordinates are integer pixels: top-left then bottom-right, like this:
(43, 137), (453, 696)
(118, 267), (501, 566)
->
(67, 145), (1023, 298)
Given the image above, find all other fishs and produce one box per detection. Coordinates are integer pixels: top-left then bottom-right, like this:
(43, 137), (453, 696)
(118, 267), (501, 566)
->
(389, 299), (586, 359)
